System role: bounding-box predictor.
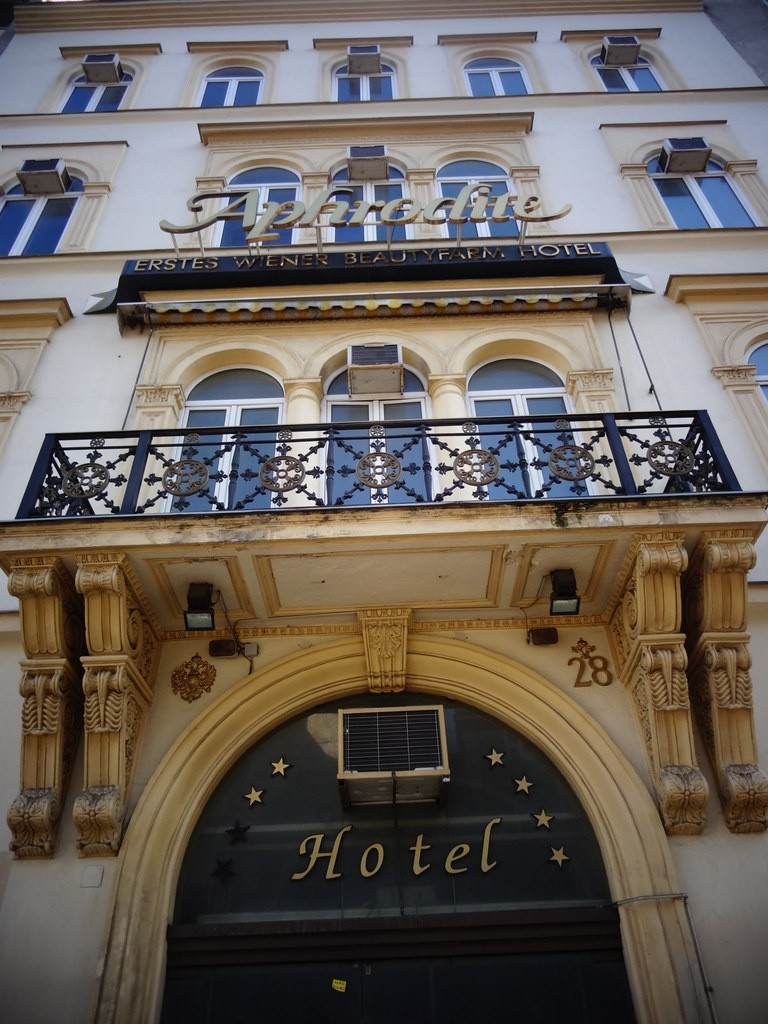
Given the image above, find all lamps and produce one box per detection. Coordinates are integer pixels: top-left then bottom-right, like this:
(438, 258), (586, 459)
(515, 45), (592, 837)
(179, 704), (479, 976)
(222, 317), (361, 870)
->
(183, 582), (216, 631)
(549, 568), (581, 615)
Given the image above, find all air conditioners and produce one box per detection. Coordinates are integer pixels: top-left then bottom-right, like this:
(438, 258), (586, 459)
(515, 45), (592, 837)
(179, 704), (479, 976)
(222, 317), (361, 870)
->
(348, 46), (382, 75)
(336, 704), (451, 808)
(17, 157), (72, 194)
(659, 137), (712, 173)
(347, 345), (404, 397)
(600, 36), (642, 66)
(82, 55), (123, 82)
(346, 145), (390, 181)
